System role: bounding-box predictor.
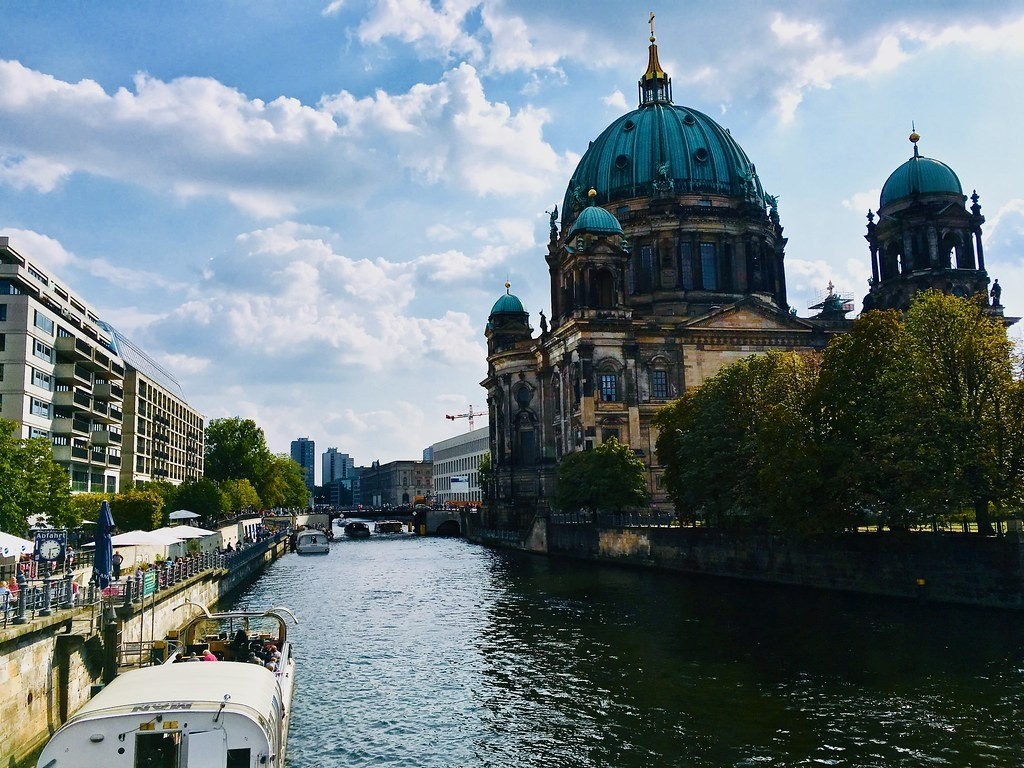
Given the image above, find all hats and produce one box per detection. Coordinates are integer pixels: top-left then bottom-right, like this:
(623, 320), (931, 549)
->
(68, 547), (72, 549)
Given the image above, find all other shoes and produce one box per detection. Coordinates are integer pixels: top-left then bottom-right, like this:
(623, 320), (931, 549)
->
(118, 576), (119, 579)
(115, 580), (117, 581)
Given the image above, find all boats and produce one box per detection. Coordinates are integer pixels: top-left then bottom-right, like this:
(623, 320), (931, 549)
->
(36, 597), (299, 768)
(331, 518), (341, 523)
(338, 518), (352, 527)
(375, 520), (403, 534)
(343, 523), (371, 538)
(296, 528), (330, 555)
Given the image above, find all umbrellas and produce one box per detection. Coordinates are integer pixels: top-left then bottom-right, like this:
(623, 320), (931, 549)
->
(91, 500), (115, 615)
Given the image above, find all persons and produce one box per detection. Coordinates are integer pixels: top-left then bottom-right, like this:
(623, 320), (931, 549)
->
(354, 502), (484, 513)
(66, 545), (75, 570)
(0, 564), (25, 610)
(249, 644), (281, 672)
(113, 550), (124, 582)
(136, 504), (334, 596)
(172, 649), (218, 663)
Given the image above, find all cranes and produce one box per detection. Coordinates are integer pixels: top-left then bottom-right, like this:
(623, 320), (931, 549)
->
(446, 405), (489, 433)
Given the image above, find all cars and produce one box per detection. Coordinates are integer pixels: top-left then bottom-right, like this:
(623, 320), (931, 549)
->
(415, 504), (431, 509)
(392, 505), (410, 510)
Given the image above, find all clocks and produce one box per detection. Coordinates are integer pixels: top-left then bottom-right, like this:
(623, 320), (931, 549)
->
(40, 540), (61, 560)
